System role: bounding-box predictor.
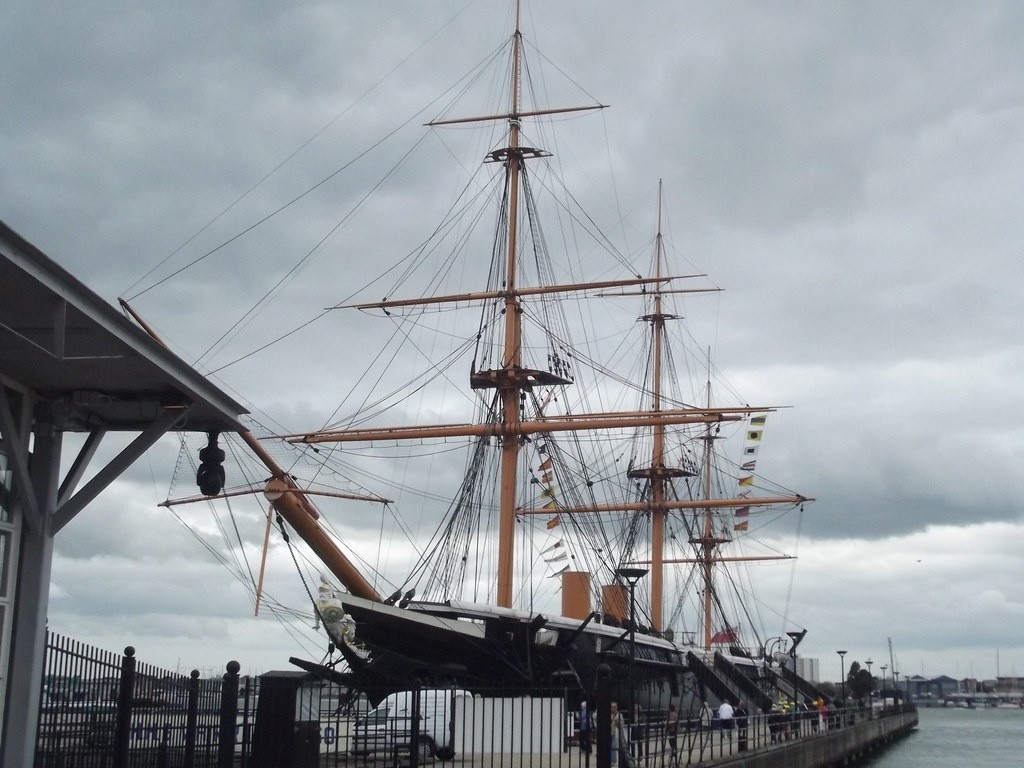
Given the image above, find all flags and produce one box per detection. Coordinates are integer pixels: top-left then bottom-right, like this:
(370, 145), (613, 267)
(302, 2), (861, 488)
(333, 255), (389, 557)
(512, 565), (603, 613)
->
(744, 445), (759, 456)
(734, 521), (748, 531)
(538, 443), (571, 578)
(738, 490), (751, 499)
(711, 626), (737, 643)
(740, 459), (756, 470)
(750, 415), (766, 426)
(739, 475), (753, 486)
(735, 507), (749, 517)
(747, 430), (762, 441)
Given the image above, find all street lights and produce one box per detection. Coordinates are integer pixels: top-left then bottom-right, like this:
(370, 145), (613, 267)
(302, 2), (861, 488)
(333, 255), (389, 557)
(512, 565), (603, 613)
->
(905, 676), (910, 703)
(865, 662), (873, 720)
(837, 651), (848, 728)
(786, 632), (803, 739)
(880, 667), (887, 713)
(614, 568), (650, 723)
(895, 672), (899, 704)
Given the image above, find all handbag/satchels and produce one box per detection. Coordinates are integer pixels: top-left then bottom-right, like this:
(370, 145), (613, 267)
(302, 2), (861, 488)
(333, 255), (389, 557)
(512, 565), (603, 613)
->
(614, 725), (632, 748)
(747, 717), (751, 725)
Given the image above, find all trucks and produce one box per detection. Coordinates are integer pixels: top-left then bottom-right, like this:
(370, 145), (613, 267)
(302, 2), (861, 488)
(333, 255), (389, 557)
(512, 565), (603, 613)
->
(351, 690), (473, 763)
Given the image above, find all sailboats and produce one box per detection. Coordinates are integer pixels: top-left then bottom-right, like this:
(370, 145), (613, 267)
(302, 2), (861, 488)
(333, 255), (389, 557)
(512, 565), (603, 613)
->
(118, 2), (782, 720)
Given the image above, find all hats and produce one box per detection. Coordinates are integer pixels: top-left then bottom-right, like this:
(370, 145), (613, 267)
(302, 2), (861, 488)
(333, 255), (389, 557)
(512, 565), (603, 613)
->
(580, 701), (586, 707)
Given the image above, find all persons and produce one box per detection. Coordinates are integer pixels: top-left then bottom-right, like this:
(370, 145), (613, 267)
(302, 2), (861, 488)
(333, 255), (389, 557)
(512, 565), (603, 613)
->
(768, 697), (866, 743)
(699, 701), (713, 727)
(668, 705), (681, 757)
(718, 700), (735, 740)
(611, 702), (624, 766)
(576, 701), (592, 755)
(633, 704), (645, 762)
(734, 700), (749, 737)
